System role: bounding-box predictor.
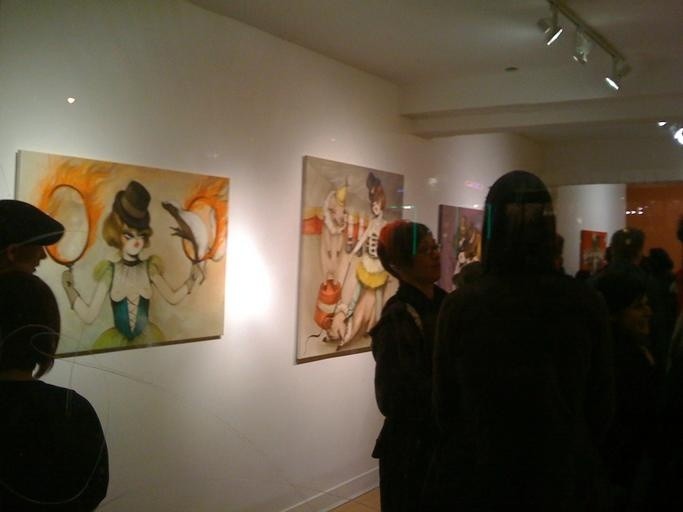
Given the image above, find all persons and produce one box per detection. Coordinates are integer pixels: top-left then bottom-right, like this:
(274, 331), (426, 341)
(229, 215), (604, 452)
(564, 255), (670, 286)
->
(429, 169), (619, 510)
(0, 270), (108, 510)
(369, 220), (450, 510)
(2, 197), (67, 276)
(556, 210), (683, 512)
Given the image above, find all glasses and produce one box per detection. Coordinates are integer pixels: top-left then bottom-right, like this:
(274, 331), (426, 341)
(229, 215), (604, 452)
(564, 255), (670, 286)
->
(411, 244), (440, 256)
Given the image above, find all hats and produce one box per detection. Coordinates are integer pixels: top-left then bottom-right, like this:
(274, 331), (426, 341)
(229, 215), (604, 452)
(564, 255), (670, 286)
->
(367, 172), (380, 201)
(115, 181), (150, 229)
(1, 199), (64, 246)
